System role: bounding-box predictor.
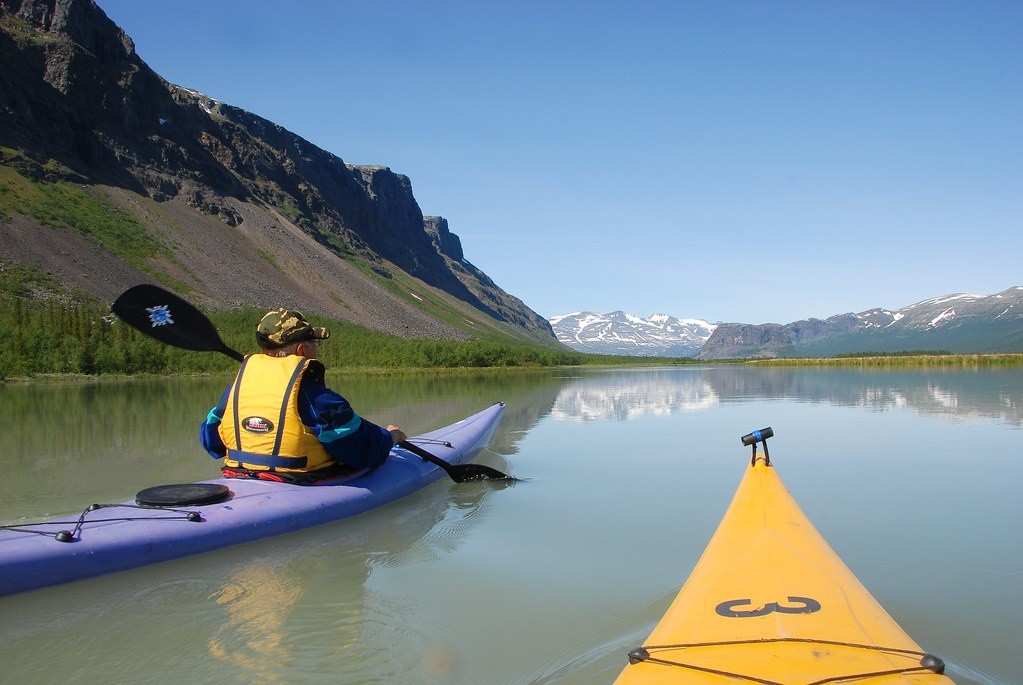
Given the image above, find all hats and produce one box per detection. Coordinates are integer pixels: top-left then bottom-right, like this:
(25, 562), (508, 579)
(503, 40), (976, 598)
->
(256, 307), (330, 350)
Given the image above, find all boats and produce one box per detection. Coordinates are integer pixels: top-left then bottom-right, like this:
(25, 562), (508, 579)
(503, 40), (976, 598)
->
(612, 428), (956, 685)
(0, 399), (510, 599)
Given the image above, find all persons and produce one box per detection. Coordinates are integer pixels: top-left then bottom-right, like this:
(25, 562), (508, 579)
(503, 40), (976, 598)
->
(200, 307), (407, 484)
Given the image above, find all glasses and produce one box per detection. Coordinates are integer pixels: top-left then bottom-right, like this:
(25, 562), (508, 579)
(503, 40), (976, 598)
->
(309, 339), (321, 346)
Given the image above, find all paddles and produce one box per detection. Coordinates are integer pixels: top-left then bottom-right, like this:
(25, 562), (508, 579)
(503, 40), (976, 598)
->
(108, 281), (512, 485)
